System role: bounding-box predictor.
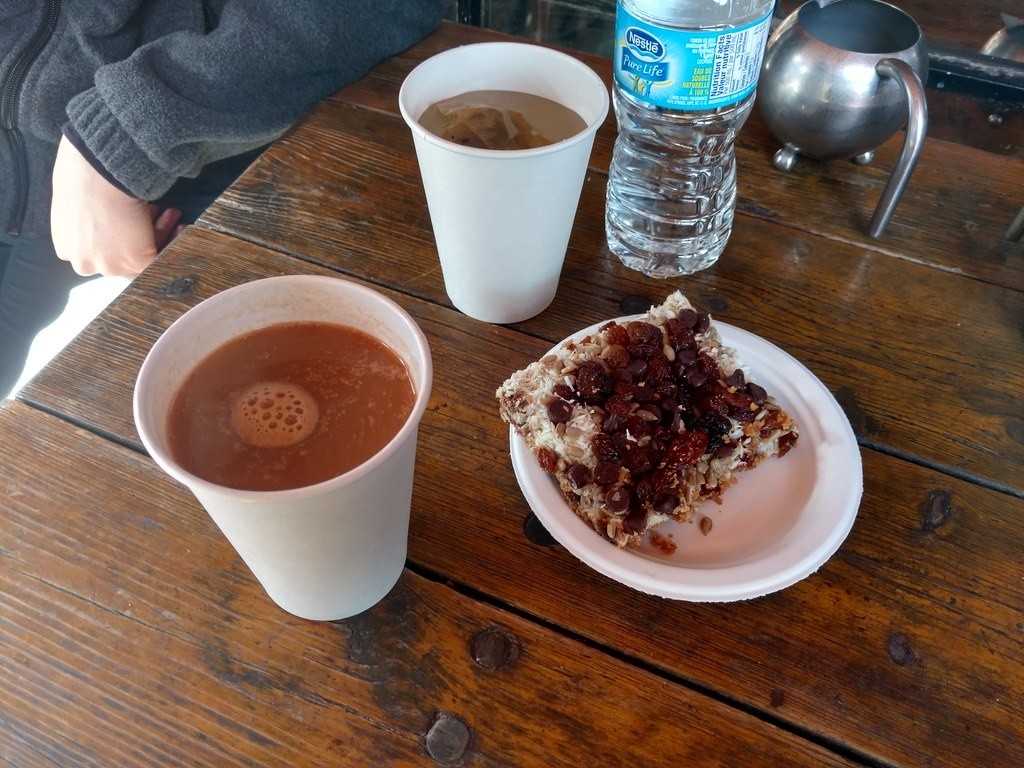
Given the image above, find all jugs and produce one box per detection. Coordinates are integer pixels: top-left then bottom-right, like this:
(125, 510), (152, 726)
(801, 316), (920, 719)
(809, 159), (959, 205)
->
(758, 1), (931, 242)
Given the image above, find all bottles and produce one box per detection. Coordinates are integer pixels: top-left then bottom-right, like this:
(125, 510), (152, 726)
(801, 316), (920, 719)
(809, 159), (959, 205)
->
(603, 0), (779, 280)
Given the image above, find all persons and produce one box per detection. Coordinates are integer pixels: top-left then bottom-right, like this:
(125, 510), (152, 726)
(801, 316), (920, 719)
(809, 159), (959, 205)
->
(0, 0), (445, 405)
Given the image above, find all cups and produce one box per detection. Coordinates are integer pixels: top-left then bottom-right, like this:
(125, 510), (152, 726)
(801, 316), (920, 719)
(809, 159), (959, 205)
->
(130, 273), (434, 623)
(398, 41), (611, 325)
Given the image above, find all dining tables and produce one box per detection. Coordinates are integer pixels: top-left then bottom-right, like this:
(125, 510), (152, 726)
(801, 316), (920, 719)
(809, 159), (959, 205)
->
(0, 17), (1024, 767)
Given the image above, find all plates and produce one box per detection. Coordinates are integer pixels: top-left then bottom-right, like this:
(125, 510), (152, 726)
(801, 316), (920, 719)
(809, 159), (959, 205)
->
(507, 309), (864, 603)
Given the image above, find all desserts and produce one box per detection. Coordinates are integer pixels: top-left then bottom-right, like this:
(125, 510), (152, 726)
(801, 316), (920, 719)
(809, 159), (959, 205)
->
(494, 288), (793, 555)
